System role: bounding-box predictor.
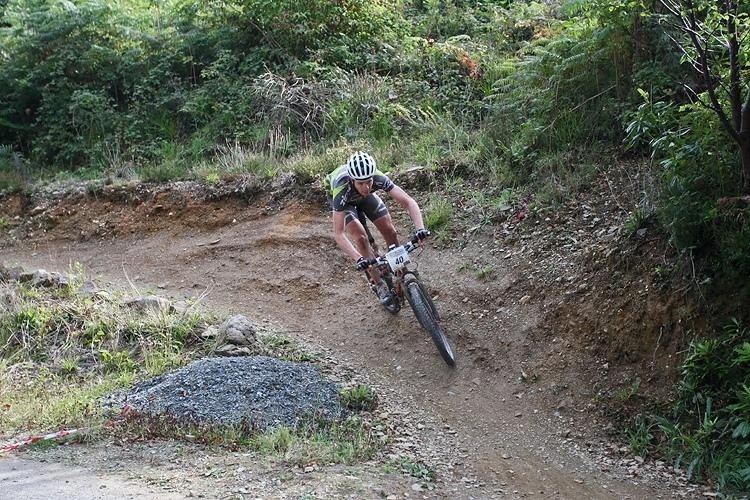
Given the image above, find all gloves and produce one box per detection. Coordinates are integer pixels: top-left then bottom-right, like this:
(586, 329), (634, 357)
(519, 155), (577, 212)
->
(356, 257), (371, 271)
(416, 229), (428, 240)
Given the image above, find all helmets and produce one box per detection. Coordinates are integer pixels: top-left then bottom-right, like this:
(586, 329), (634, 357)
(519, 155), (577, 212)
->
(347, 151), (376, 183)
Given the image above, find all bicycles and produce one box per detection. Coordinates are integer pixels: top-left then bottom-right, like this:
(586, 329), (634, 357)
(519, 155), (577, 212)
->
(355, 229), (458, 369)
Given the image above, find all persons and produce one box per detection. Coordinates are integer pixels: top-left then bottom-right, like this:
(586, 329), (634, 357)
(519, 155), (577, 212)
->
(324, 150), (430, 306)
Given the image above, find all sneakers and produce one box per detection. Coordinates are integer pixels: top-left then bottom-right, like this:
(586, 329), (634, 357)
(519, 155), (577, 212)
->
(375, 278), (392, 305)
